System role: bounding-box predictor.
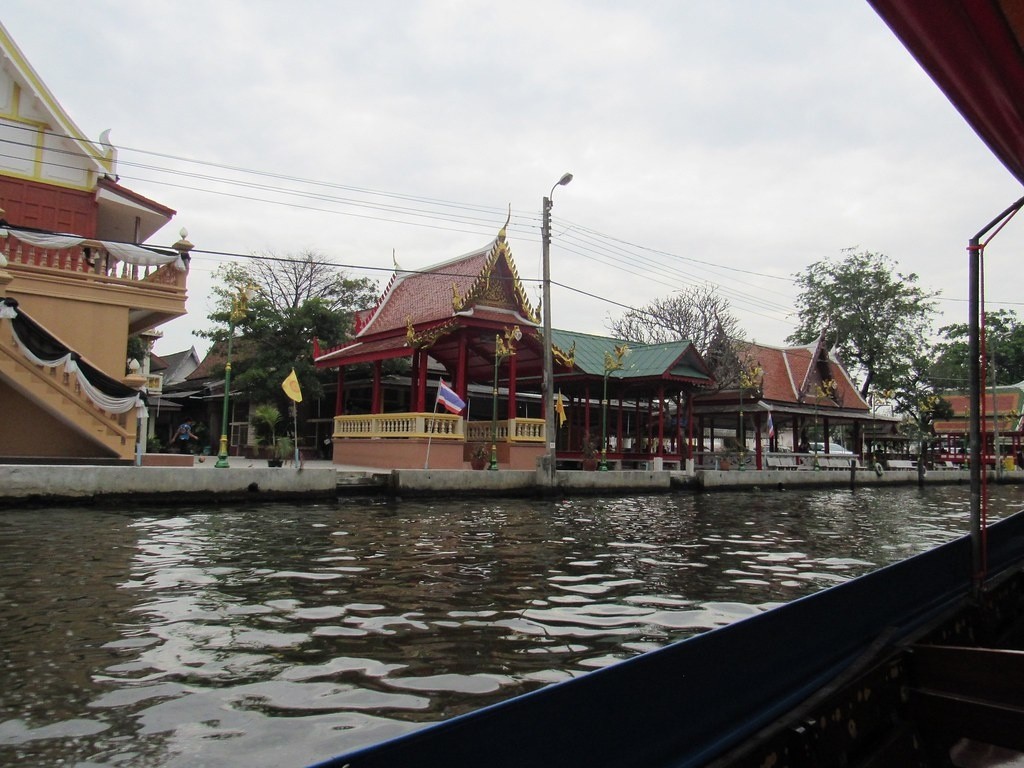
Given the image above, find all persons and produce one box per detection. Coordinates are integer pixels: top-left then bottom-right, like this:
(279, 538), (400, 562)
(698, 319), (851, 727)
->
(875, 444), (886, 467)
(169, 418), (198, 455)
(800, 437), (812, 453)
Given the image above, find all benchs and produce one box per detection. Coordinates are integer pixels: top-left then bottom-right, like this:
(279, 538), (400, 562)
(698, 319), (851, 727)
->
(767, 458), (798, 471)
(887, 460), (917, 471)
(817, 458), (867, 471)
(945, 460), (959, 470)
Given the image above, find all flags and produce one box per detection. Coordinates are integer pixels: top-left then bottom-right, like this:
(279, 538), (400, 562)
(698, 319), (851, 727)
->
(767, 414), (775, 438)
(281, 371), (302, 402)
(439, 381), (465, 413)
(556, 394), (567, 428)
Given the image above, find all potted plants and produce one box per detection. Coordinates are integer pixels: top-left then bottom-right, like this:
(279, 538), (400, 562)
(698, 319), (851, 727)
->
(471, 434), (488, 470)
(249, 404), (295, 467)
(715, 453), (731, 471)
(146, 432), (160, 453)
(577, 434), (602, 471)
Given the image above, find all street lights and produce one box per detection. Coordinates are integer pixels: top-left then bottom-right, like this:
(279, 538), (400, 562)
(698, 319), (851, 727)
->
(543, 173), (575, 470)
(870, 388), (897, 468)
(740, 362), (764, 469)
(815, 378), (837, 470)
(918, 395), (939, 469)
(216, 276), (260, 468)
(487, 326), (522, 470)
(1000, 408), (1020, 467)
(598, 344), (632, 470)
